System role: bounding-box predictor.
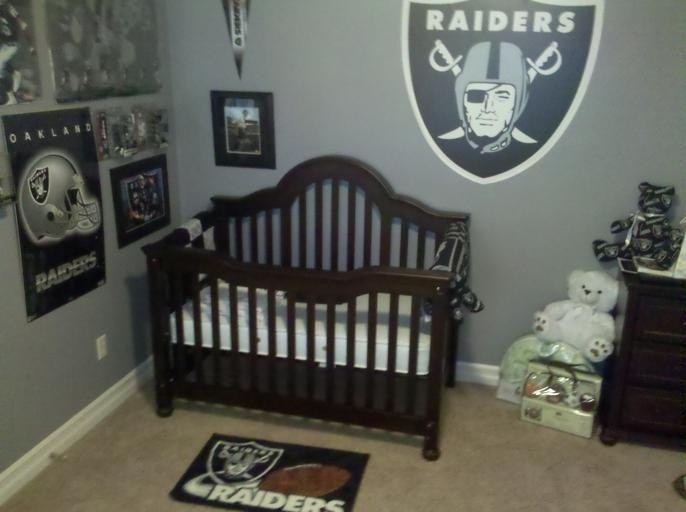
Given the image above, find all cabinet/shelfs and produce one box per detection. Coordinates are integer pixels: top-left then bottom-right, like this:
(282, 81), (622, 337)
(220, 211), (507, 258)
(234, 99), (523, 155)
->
(596, 265), (685, 452)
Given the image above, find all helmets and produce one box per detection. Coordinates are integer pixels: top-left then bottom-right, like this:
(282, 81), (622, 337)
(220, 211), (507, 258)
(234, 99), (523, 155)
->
(19, 154), (98, 240)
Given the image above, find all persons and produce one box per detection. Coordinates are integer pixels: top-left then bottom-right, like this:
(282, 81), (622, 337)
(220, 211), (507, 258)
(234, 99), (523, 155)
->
(229, 105), (260, 152)
(436, 40), (538, 178)
(129, 173), (159, 222)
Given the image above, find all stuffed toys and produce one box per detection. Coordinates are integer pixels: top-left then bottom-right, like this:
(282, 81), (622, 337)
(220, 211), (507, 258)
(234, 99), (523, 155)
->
(532, 269), (620, 366)
(592, 180), (684, 271)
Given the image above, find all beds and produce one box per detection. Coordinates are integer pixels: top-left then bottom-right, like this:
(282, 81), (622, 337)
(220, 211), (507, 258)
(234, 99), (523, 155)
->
(137, 156), (471, 463)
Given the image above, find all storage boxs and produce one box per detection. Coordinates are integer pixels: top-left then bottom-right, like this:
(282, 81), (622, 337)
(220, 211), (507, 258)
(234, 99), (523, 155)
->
(520, 363), (604, 441)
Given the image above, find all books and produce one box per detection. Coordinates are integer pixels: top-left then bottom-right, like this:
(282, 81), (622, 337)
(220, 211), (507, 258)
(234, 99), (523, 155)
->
(616, 255), (637, 275)
(633, 257), (675, 278)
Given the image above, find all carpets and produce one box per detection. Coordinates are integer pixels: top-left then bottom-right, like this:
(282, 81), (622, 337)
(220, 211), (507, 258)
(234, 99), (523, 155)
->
(166, 430), (372, 511)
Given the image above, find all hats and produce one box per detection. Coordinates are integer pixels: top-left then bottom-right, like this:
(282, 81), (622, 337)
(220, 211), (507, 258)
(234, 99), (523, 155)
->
(453, 41), (526, 153)
(226, 452), (254, 476)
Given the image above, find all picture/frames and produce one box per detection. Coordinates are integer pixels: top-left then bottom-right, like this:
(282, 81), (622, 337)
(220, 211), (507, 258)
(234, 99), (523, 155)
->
(208, 88), (279, 171)
(109, 154), (170, 248)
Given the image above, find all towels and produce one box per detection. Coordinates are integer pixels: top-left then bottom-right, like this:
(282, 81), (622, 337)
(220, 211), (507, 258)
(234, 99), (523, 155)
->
(420, 223), (483, 326)
